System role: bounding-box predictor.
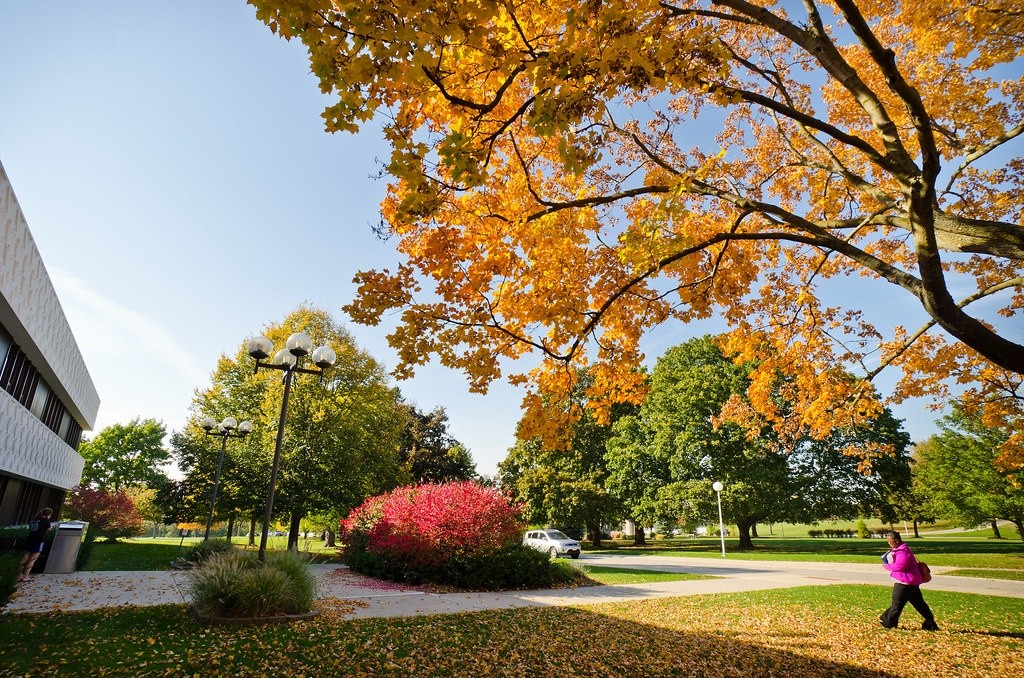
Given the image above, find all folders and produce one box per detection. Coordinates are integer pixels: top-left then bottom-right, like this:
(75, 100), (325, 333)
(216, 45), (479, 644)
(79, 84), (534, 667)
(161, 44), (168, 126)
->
(881, 549), (892, 564)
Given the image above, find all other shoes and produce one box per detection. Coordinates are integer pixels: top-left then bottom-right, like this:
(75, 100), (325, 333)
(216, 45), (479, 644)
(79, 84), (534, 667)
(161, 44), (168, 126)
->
(879, 622), (897, 629)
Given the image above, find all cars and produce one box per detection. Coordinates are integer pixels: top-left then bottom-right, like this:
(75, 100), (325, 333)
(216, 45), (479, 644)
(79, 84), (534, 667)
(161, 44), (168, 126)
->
(667, 529), (682, 535)
(246, 531), (301, 536)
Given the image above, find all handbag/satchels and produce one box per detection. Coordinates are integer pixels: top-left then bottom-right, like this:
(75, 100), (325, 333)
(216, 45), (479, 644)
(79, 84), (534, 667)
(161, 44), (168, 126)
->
(918, 562), (931, 583)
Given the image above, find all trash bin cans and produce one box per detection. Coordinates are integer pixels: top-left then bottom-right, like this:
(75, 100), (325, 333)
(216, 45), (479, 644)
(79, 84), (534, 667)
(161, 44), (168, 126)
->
(44, 524), (84, 574)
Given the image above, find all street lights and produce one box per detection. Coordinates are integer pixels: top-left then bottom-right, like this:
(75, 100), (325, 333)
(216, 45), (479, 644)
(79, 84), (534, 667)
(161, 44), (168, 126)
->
(248, 331), (336, 562)
(200, 418), (253, 538)
(713, 482), (726, 557)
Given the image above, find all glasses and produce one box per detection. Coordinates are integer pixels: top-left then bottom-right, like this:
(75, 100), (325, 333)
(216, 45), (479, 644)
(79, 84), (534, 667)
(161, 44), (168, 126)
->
(888, 537), (897, 545)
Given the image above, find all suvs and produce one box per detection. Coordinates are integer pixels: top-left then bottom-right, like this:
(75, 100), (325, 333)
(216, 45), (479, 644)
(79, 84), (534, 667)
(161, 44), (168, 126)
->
(523, 529), (581, 559)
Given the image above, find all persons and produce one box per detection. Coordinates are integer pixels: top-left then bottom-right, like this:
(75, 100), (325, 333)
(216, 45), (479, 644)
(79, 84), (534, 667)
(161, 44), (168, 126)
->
(18, 508), (53, 581)
(879, 531), (939, 630)
